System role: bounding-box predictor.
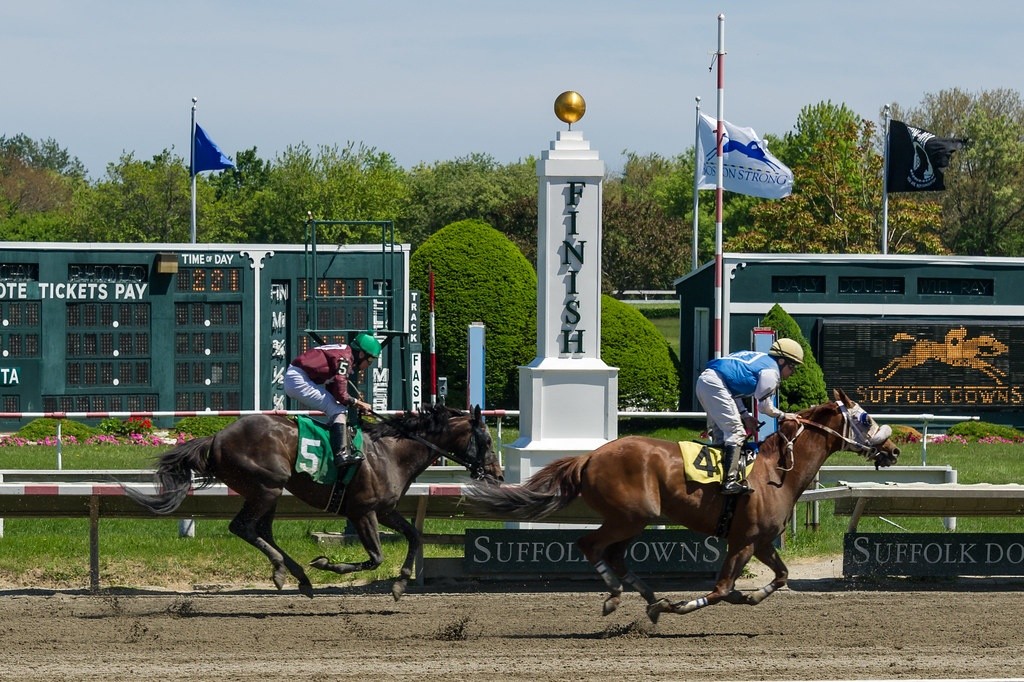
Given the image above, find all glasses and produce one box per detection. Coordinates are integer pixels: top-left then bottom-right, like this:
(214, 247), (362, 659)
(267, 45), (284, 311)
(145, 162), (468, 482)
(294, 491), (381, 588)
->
(365, 358), (373, 365)
(786, 360), (800, 369)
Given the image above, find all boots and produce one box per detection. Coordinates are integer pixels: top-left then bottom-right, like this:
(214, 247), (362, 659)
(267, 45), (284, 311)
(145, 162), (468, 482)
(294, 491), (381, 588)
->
(720, 445), (755, 495)
(332, 422), (365, 466)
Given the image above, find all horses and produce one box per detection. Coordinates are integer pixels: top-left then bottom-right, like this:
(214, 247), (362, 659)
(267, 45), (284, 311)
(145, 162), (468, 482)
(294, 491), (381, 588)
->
(120, 406), (503, 602)
(467, 386), (900, 623)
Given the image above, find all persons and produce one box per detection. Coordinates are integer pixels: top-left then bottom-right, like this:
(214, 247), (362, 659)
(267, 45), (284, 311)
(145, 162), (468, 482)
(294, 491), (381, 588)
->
(286, 334), (380, 469)
(697, 338), (804, 493)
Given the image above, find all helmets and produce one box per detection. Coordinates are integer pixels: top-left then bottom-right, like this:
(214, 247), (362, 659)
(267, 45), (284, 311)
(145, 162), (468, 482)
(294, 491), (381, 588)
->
(351, 333), (382, 358)
(769, 338), (804, 365)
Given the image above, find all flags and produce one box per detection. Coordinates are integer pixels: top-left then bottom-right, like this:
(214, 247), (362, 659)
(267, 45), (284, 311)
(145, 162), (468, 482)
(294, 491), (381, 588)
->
(887, 118), (968, 193)
(192, 120), (234, 178)
(694, 113), (791, 199)
